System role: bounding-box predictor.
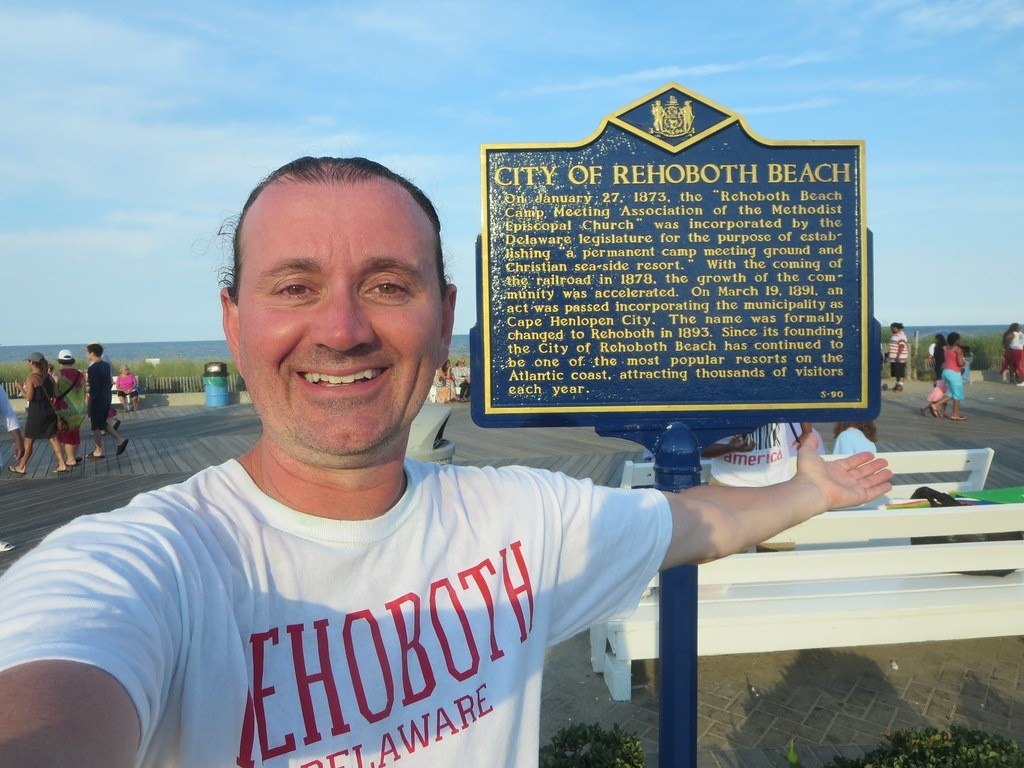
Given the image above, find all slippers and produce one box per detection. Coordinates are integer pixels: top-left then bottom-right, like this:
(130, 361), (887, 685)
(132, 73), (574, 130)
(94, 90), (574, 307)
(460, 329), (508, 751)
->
(116, 439), (128, 455)
(8, 465), (27, 474)
(86, 451), (105, 459)
(53, 469), (66, 474)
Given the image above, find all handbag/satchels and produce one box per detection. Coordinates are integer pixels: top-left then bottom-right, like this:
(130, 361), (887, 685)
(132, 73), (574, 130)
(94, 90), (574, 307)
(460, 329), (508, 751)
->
(908, 486), (1022, 577)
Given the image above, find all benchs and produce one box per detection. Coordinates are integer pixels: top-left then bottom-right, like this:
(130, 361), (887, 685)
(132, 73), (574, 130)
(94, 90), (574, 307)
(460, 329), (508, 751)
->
(111, 375), (139, 411)
(590, 447), (1024, 700)
(430, 370), (461, 403)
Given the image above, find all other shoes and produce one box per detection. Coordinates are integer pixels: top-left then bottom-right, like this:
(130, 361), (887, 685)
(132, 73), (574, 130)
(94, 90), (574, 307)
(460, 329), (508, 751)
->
(113, 420), (121, 430)
(893, 384), (903, 391)
(930, 404), (938, 417)
(90, 430), (94, 436)
(951, 415), (967, 420)
(101, 429), (106, 436)
(921, 406), (926, 416)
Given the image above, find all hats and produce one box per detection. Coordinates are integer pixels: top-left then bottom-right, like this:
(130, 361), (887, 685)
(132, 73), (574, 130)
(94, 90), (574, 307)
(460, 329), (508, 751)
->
(59, 349), (72, 360)
(26, 351), (44, 362)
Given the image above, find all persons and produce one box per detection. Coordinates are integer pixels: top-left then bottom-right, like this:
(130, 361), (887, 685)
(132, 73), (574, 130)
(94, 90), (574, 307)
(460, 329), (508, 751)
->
(0, 156), (893, 767)
(832, 419), (877, 456)
(702, 421), (812, 552)
(450, 357), (472, 402)
(52, 349), (86, 473)
(47, 363), (53, 376)
(116, 365), (138, 413)
(90, 353), (121, 436)
(429, 359), (461, 404)
(929, 332), (967, 420)
(921, 335), (949, 418)
(87, 343), (128, 458)
(0, 386), (25, 551)
(888, 322), (909, 391)
(7, 351), (69, 475)
(1002, 322), (1024, 386)
(962, 346), (973, 385)
